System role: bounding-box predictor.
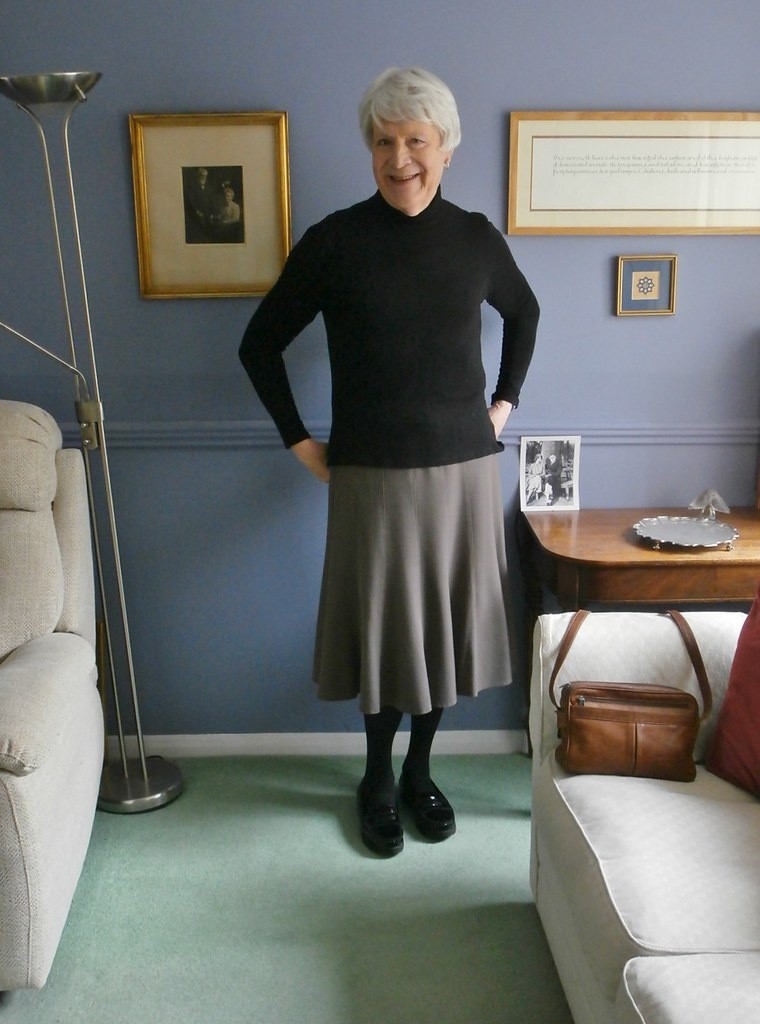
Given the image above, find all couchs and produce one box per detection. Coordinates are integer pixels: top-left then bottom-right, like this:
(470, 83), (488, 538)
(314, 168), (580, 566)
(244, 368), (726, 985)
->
(2, 398), (109, 1008)
(530, 609), (759, 1024)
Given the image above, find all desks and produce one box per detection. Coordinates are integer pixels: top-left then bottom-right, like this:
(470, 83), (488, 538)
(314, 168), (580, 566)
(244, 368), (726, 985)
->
(516, 506), (760, 612)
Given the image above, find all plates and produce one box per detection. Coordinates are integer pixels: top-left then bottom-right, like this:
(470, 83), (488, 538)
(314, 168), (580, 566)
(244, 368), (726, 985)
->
(632, 516), (740, 551)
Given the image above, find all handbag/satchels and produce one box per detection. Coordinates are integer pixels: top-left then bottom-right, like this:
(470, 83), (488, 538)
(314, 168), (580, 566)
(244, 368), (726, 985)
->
(548, 609), (714, 784)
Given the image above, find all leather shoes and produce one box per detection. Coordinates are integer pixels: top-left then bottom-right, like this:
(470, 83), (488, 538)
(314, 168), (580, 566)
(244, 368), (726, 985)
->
(399, 773), (456, 838)
(357, 779), (403, 856)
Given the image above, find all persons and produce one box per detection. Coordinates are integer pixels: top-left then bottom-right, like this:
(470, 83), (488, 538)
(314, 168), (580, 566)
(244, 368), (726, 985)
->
(526, 454), (545, 503)
(217, 189), (240, 223)
(186, 168), (219, 241)
(546, 454), (562, 505)
(240, 66), (541, 859)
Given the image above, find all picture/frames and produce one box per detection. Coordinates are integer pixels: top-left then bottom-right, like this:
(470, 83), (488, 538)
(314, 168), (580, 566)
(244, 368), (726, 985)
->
(128, 113), (293, 300)
(616, 255), (676, 315)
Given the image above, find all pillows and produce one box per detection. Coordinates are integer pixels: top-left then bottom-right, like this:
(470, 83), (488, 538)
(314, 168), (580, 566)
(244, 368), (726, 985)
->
(701, 589), (759, 798)
